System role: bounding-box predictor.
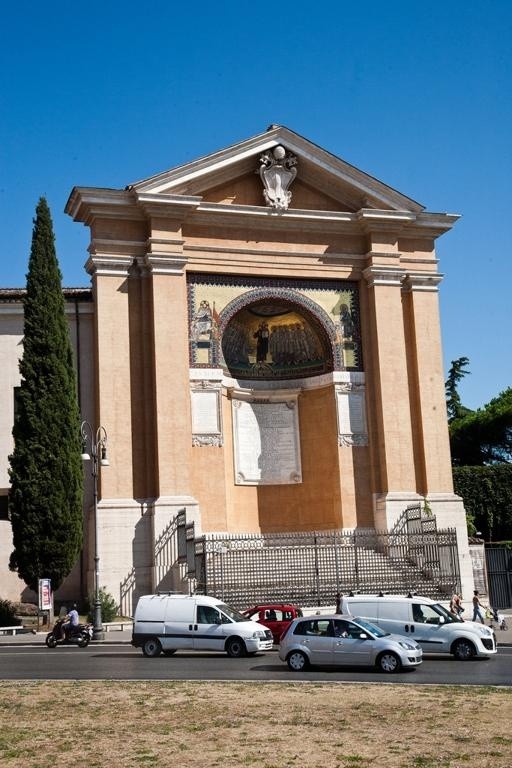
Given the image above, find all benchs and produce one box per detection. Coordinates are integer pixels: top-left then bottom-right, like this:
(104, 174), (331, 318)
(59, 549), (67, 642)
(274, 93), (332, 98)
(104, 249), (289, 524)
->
(0, 625), (24, 635)
(101, 621), (132, 631)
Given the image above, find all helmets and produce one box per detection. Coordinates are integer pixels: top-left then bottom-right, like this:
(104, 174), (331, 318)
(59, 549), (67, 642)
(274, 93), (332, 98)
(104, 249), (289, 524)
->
(71, 603), (78, 610)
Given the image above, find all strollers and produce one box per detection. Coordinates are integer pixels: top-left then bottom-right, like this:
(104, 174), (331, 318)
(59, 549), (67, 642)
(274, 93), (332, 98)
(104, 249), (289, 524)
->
(484, 604), (508, 631)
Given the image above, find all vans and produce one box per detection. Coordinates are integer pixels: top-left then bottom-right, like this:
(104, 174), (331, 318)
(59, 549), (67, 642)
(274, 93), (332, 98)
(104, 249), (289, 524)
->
(338, 595), (498, 661)
(131, 591), (275, 658)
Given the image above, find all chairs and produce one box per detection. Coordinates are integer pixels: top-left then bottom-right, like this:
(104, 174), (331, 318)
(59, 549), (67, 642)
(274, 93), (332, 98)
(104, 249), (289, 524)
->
(326, 624), (335, 637)
(266, 611), (277, 621)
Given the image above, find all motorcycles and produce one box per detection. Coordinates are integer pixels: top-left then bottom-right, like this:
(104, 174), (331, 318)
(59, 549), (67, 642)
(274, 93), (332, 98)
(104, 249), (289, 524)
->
(45, 605), (95, 648)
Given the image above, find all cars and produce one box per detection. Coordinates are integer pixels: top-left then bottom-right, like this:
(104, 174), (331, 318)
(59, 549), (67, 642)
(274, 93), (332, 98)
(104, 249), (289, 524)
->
(277, 612), (424, 674)
(241, 605), (304, 644)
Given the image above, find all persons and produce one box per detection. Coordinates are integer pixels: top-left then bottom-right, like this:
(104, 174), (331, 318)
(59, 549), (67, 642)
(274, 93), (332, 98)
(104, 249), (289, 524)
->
(194, 300), (217, 337)
(493, 608), (507, 631)
(338, 302), (355, 340)
(472, 590), (487, 624)
(55, 602), (80, 642)
(449, 592), (465, 620)
(222, 320), (325, 364)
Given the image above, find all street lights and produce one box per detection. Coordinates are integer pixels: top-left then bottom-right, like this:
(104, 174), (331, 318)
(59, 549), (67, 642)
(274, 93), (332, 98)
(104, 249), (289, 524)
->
(79, 419), (112, 640)
